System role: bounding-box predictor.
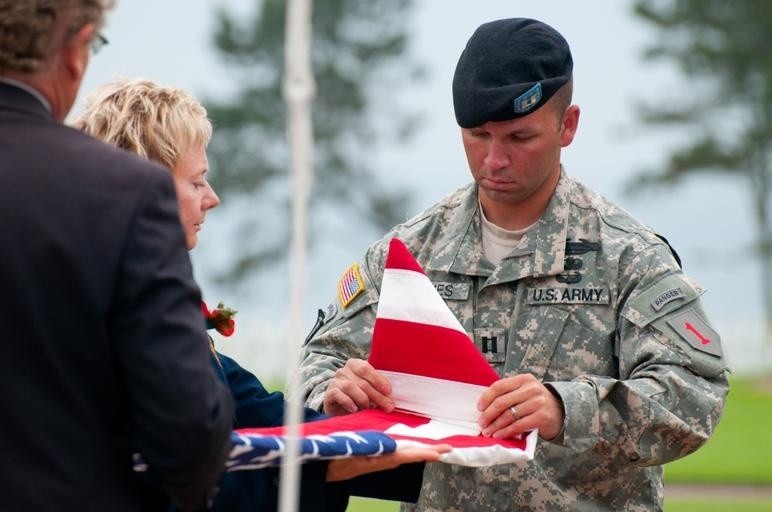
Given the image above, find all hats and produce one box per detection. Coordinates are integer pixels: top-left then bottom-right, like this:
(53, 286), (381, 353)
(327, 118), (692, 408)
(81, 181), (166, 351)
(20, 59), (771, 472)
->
(452, 18), (576, 128)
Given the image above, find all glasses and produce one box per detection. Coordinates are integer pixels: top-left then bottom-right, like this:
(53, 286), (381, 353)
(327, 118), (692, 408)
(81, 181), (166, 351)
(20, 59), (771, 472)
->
(64, 18), (112, 55)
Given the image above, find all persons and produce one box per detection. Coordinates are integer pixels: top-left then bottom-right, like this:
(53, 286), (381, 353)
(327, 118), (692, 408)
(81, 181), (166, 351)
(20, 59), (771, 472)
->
(293, 17), (734, 512)
(0, 0), (237, 512)
(68, 75), (455, 511)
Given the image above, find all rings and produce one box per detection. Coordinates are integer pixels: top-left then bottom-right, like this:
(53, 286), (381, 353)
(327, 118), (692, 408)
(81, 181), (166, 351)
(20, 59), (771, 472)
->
(510, 406), (520, 421)
